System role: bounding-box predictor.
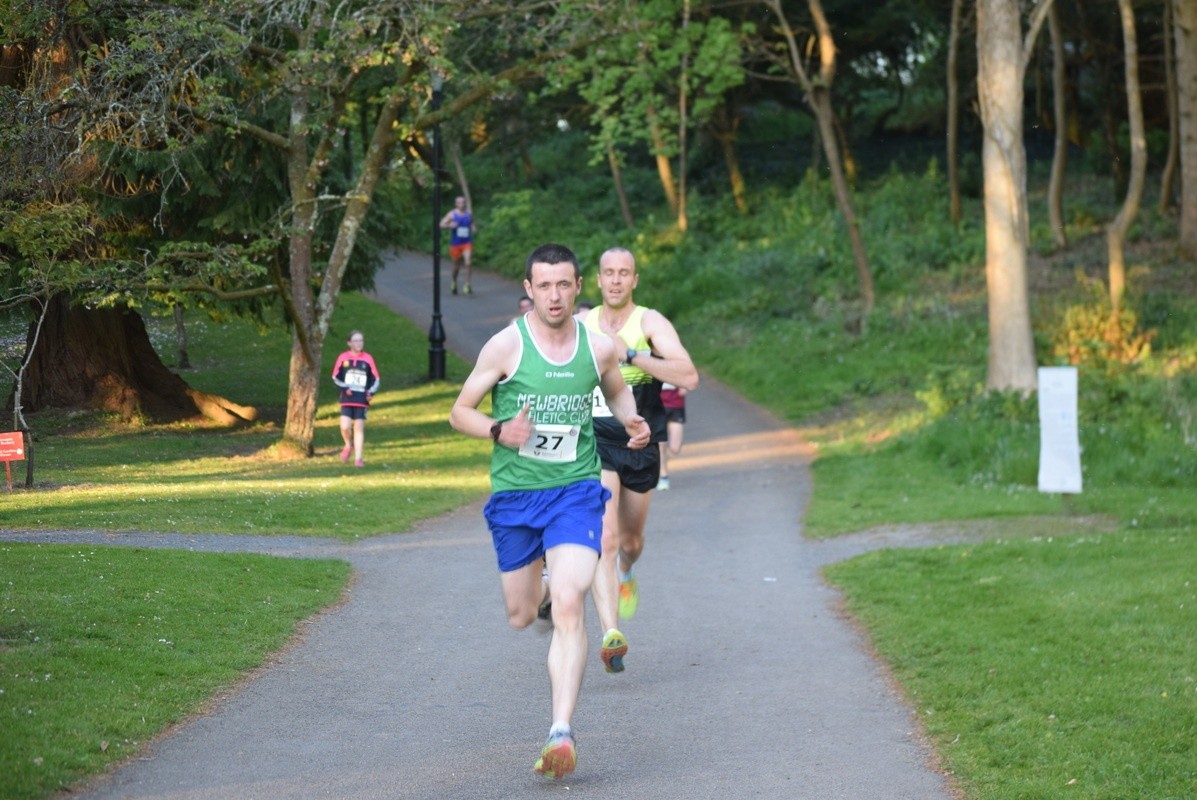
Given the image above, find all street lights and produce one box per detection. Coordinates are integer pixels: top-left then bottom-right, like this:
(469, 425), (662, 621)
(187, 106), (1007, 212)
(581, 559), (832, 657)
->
(419, 61), (455, 379)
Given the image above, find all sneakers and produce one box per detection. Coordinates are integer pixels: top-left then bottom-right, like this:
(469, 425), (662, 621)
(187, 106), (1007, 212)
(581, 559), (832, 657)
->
(537, 557), (553, 619)
(532, 730), (576, 782)
(601, 629), (628, 673)
(619, 572), (639, 619)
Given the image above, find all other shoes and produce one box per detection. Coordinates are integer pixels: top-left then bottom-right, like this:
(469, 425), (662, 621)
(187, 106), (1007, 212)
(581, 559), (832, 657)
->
(354, 460), (362, 467)
(341, 446), (351, 462)
(464, 285), (472, 293)
(656, 478), (669, 490)
(452, 289), (457, 294)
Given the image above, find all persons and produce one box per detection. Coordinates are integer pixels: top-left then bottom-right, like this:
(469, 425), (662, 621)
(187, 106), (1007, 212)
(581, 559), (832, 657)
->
(577, 301), (593, 314)
(509, 296), (534, 325)
(331, 328), (381, 467)
(449, 243), (653, 781)
(536, 551), (553, 630)
(572, 247), (700, 670)
(655, 381), (688, 492)
(438, 195), (475, 295)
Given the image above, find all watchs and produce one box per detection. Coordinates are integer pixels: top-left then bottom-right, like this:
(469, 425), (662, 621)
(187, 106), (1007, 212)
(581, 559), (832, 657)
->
(490, 416), (513, 442)
